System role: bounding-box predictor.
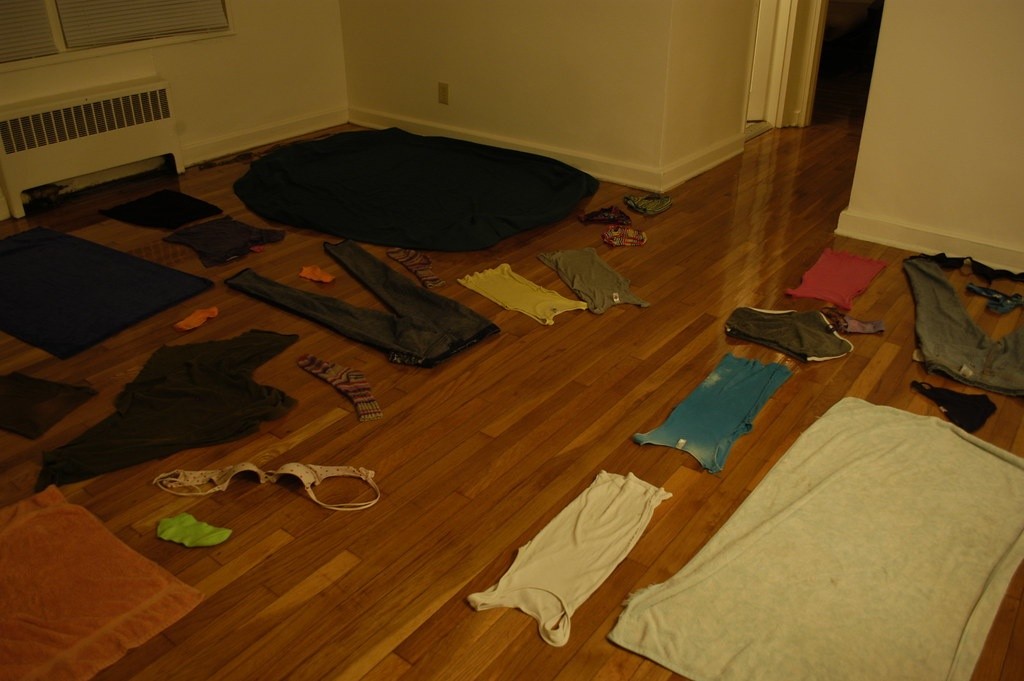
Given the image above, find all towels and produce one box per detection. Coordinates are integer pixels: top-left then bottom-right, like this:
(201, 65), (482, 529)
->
(604, 394), (1024, 681)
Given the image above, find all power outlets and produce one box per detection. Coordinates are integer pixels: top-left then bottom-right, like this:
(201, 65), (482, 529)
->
(439, 82), (448, 105)
